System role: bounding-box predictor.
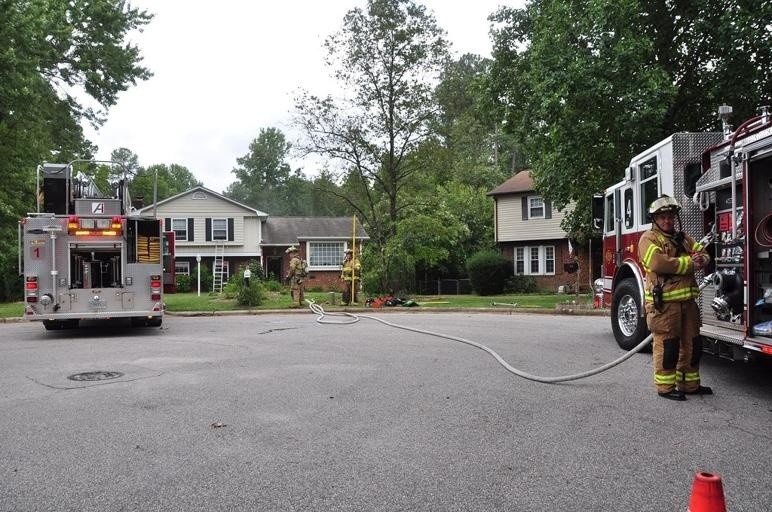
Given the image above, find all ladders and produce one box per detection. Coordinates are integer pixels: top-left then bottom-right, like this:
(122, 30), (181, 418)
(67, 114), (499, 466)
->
(213, 241), (224, 294)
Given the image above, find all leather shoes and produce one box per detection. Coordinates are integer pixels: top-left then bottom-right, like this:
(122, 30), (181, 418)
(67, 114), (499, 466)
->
(686, 386), (713, 394)
(658, 389), (685, 400)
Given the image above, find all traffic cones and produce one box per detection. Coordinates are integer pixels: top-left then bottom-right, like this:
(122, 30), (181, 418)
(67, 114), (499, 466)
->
(686, 469), (726, 512)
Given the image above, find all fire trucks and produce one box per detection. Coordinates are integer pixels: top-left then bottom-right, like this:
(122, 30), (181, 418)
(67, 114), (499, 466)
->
(16, 156), (176, 330)
(591, 99), (772, 371)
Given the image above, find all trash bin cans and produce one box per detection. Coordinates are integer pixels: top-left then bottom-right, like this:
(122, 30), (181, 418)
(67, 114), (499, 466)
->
(593, 278), (605, 309)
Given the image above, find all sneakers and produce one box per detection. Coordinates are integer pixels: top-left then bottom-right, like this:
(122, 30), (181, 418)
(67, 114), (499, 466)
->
(340, 301), (358, 306)
(289, 302), (302, 308)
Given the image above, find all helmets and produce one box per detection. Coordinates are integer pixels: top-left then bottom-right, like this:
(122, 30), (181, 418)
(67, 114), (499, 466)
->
(285, 246), (297, 253)
(648, 195), (681, 216)
(344, 249), (353, 254)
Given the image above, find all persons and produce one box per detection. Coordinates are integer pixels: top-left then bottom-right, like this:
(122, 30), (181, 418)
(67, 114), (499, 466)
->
(283, 246), (309, 309)
(338, 249), (361, 306)
(636, 193), (713, 403)
(244, 264), (252, 287)
